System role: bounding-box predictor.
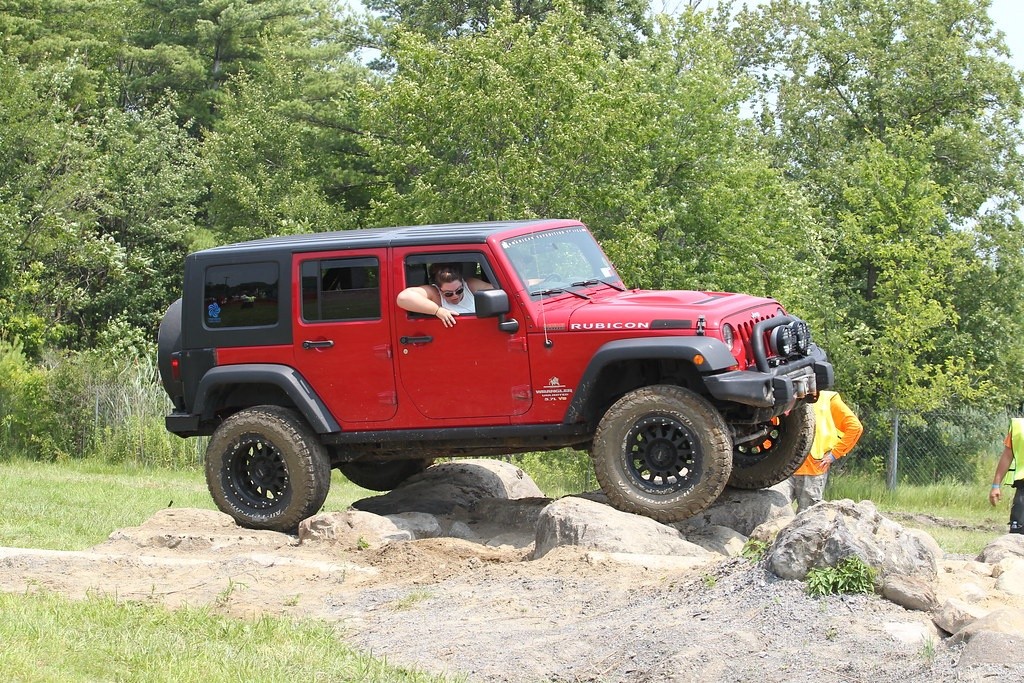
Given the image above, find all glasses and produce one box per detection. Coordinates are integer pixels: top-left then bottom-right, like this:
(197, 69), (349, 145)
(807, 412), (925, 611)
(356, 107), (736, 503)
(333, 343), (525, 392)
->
(440, 287), (463, 297)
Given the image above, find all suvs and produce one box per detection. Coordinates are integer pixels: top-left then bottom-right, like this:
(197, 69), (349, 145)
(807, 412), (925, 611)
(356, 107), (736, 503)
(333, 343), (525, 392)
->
(157, 219), (834, 523)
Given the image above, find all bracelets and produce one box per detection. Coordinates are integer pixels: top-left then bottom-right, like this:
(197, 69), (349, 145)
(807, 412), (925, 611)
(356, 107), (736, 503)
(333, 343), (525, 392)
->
(991, 484), (999, 488)
(829, 453), (836, 461)
(434, 306), (441, 315)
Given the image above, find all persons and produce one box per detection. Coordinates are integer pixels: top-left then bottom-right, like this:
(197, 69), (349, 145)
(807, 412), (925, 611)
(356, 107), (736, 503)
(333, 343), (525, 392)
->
(395, 263), (494, 328)
(990, 418), (1024, 536)
(768, 389), (863, 514)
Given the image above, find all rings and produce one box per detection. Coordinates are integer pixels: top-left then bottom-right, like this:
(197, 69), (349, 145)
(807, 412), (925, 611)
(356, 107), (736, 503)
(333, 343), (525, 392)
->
(446, 319), (449, 322)
(449, 316), (452, 319)
